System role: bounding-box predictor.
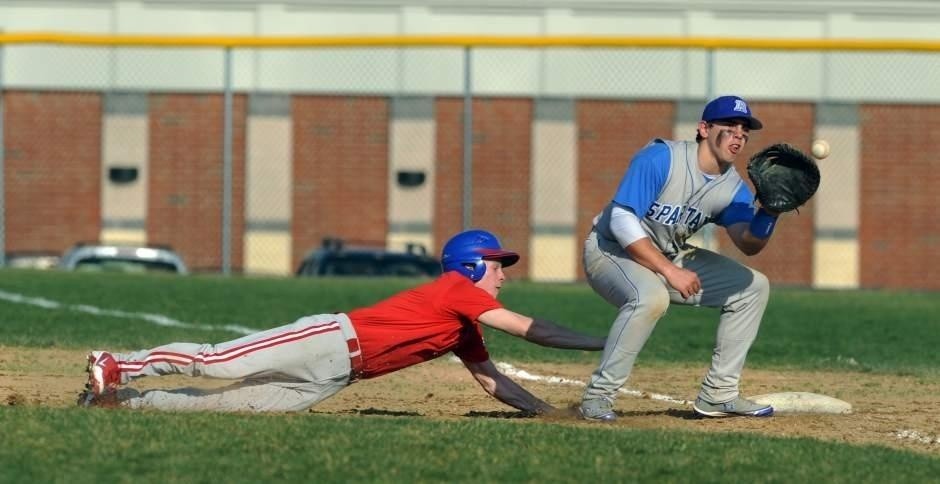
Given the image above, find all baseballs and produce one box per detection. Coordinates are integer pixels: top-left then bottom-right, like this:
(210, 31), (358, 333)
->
(810, 139), (830, 159)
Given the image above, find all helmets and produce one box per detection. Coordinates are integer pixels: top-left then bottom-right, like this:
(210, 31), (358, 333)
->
(440, 229), (522, 281)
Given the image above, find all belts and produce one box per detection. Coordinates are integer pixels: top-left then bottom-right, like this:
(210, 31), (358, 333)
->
(333, 311), (368, 386)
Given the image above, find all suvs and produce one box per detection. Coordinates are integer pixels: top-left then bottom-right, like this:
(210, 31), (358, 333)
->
(298, 239), (440, 281)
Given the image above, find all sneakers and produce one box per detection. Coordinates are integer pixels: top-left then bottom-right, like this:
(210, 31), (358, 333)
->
(77, 350), (122, 408)
(574, 394), (620, 424)
(691, 389), (776, 420)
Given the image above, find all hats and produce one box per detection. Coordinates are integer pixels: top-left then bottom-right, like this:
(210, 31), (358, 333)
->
(700, 93), (765, 133)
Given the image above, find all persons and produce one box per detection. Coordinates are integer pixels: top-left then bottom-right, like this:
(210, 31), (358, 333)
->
(74, 227), (608, 420)
(573, 89), (822, 424)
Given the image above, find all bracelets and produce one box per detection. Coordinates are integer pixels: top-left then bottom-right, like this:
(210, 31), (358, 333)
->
(747, 207), (780, 242)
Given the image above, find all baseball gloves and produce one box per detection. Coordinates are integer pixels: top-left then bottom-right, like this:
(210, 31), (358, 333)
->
(747, 142), (819, 212)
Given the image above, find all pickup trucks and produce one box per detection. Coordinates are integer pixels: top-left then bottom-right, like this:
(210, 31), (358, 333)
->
(58, 243), (187, 275)
